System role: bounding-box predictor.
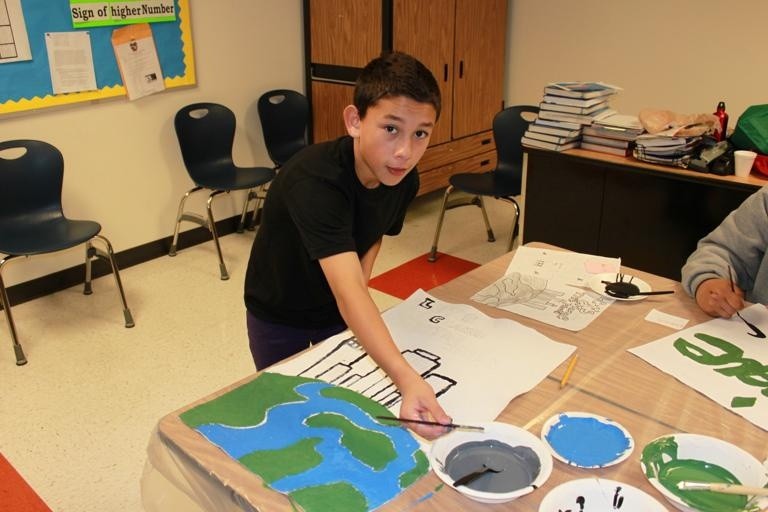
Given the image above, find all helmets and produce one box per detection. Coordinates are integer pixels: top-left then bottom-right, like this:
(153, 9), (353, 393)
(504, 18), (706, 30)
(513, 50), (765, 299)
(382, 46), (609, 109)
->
(536, 477), (668, 512)
(586, 271), (652, 303)
(539, 411), (634, 470)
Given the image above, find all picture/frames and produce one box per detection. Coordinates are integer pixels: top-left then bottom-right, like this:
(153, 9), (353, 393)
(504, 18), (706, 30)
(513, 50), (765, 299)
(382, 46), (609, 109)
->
(517, 139), (768, 283)
(158, 240), (768, 510)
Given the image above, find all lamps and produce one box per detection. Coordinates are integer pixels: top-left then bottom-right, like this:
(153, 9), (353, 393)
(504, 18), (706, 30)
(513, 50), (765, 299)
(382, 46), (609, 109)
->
(167, 101), (275, 282)
(240, 88), (314, 233)
(0, 138), (135, 366)
(427, 105), (541, 264)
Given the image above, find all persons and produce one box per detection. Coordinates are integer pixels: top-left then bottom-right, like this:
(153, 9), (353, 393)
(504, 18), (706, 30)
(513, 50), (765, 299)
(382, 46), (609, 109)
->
(681, 182), (767, 319)
(244, 53), (455, 440)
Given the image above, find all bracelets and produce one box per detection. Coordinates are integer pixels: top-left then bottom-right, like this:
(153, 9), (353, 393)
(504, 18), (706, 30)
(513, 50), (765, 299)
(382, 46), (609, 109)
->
(428, 420), (553, 505)
(640, 434), (767, 512)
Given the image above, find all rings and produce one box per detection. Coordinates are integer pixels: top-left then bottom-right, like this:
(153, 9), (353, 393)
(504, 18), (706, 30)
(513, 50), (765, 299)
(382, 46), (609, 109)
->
(559, 353), (580, 388)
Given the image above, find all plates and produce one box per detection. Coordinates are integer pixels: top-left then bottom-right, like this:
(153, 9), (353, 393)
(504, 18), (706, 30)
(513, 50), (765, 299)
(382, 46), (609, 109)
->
(302, 0), (511, 198)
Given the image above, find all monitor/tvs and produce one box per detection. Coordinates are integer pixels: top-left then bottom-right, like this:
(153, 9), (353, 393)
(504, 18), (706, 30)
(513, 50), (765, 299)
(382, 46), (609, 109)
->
(111, 23), (165, 101)
(581, 110), (646, 157)
(633, 132), (704, 169)
(45, 31), (96, 94)
(521, 80), (623, 151)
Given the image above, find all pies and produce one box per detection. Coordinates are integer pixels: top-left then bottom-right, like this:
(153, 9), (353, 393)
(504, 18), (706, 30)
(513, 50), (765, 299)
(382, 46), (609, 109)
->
(728, 265), (734, 292)
(629, 291), (674, 296)
(677, 481), (768, 496)
(376, 415), (485, 430)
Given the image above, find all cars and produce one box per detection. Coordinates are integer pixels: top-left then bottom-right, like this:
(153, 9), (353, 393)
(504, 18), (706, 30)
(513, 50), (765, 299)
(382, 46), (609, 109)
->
(733, 149), (759, 179)
